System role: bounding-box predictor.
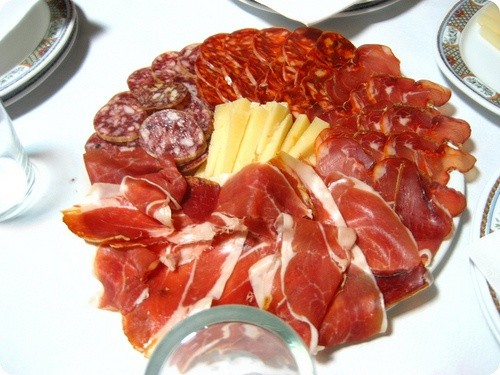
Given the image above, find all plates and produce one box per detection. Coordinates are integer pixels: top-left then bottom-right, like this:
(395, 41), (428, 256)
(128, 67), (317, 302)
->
(435, 0), (500, 117)
(476, 174), (500, 344)
(0, 0), (79, 108)
(236, 0), (400, 18)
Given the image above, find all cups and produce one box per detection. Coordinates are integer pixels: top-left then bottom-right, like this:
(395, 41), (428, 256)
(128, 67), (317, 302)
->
(144, 303), (314, 375)
(0, 98), (36, 221)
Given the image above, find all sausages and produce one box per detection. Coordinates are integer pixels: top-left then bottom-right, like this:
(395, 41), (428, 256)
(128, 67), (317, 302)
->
(84, 42), (216, 172)
